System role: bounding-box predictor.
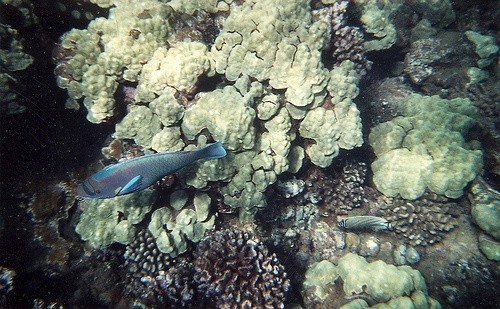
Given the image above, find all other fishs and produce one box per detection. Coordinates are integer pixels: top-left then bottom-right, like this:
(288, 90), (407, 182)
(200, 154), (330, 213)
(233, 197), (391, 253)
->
(337, 216), (397, 231)
(76, 140), (228, 199)
